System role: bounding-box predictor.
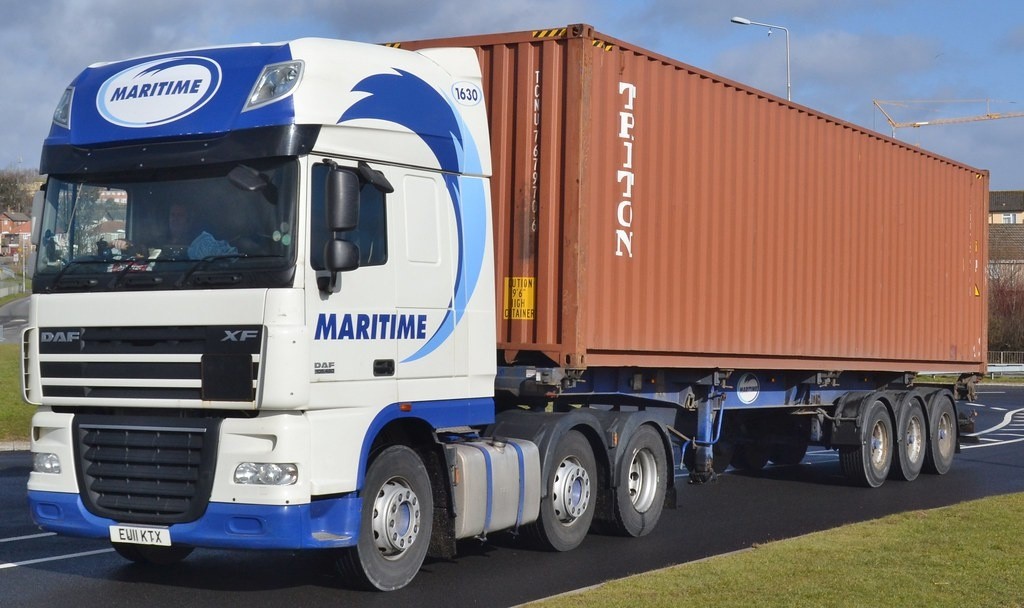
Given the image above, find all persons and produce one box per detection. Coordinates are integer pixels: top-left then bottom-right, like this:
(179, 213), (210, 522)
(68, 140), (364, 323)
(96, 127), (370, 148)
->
(105, 199), (208, 266)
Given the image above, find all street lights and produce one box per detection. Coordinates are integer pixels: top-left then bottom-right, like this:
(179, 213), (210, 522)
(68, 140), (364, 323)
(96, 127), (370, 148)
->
(731, 17), (791, 101)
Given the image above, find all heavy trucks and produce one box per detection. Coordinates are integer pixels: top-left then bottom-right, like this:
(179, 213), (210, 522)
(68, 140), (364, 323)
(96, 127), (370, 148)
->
(19, 23), (990, 594)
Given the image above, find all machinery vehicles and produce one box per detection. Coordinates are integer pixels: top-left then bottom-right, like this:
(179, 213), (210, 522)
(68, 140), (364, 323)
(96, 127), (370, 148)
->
(872, 98), (1024, 147)
